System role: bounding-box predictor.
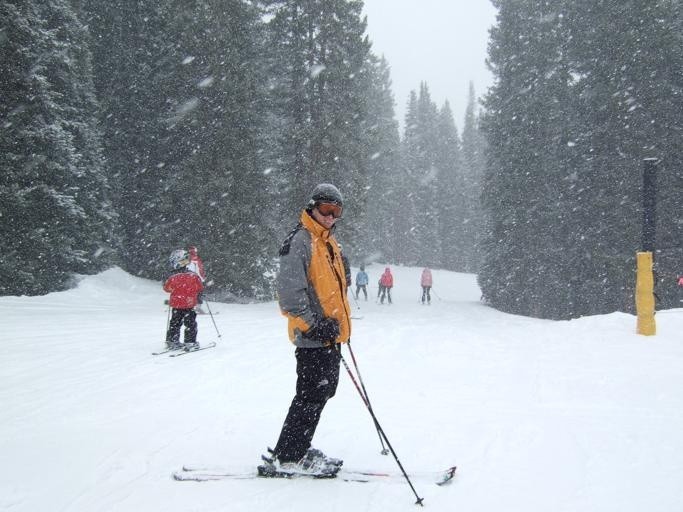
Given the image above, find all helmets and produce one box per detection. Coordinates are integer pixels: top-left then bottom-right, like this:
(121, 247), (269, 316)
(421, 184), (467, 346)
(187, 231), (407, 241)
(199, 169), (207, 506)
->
(169, 250), (189, 262)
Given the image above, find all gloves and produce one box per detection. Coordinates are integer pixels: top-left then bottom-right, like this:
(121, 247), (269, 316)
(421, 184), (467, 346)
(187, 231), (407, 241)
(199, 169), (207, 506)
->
(316, 317), (340, 341)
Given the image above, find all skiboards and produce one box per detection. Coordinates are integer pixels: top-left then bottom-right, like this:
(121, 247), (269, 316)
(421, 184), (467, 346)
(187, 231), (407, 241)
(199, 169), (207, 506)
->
(173, 466), (456, 485)
(152, 342), (216, 357)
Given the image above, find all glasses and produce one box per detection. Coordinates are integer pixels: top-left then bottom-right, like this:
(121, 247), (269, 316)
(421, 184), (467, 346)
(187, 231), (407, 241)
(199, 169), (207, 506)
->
(317, 204), (343, 218)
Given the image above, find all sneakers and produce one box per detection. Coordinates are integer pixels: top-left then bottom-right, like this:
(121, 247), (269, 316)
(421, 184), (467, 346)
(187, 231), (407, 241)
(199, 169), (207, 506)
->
(169, 342), (183, 348)
(185, 342), (200, 351)
(268, 449), (340, 476)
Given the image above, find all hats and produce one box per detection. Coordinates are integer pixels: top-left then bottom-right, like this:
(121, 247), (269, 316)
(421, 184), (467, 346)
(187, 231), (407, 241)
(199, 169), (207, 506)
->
(310, 184), (344, 204)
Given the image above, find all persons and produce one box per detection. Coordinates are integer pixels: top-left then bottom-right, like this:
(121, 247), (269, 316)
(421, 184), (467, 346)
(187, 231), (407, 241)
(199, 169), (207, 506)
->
(421, 266), (433, 303)
(270, 183), (350, 479)
(163, 246), (214, 352)
(355, 263), (394, 304)
